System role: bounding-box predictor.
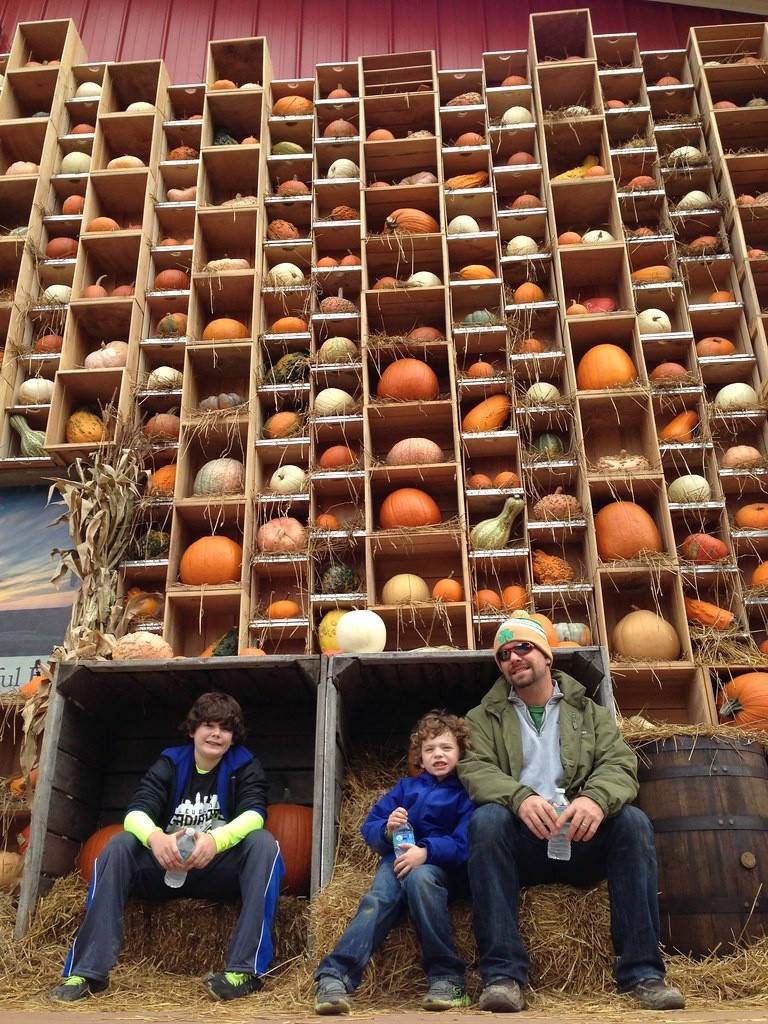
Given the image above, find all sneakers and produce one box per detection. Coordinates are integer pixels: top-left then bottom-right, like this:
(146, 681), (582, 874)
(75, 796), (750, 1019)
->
(51, 974), (108, 1002)
(313, 976), (351, 1012)
(204, 971), (264, 1002)
(422, 981), (471, 1011)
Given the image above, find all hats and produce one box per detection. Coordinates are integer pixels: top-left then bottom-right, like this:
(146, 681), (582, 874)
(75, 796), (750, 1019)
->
(493, 609), (554, 673)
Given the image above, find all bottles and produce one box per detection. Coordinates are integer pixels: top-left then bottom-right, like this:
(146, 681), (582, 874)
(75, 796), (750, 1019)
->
(547, 788), (571, 861)
(393, 816), (417, 889)
(163, 828), (196, 889)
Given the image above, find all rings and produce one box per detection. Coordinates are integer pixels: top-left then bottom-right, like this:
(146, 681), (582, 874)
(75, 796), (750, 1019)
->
(581, 822), (588, 828)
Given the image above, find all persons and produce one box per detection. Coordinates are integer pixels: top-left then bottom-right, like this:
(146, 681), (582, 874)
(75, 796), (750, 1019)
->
(46, 687), (286, 1003)
(313, 707), (477, 1014)
(455, 618), (686, 1012)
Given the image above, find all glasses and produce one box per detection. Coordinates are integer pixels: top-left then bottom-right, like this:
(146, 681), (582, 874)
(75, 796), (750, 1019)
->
(496, 642), (533, 662)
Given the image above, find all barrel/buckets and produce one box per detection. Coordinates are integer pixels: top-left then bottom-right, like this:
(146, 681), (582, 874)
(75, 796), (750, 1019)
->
(628, 738), (768, 962)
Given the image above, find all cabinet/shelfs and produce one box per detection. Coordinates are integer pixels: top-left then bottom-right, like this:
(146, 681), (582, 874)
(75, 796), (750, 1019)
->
(0, 8), (768, 978)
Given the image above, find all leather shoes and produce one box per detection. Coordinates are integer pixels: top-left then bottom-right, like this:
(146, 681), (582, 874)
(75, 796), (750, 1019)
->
(633, 978), (685, 1010)
(479, 977), (525, 1011)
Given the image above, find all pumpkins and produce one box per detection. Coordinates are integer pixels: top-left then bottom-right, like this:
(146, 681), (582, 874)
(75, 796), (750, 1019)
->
(0, 52), (768, 734)
(264, 788), (312, 898)
(0, 765), (38, 895)
(408, 737), (426, 777)
(77, 824), (124, 884)
